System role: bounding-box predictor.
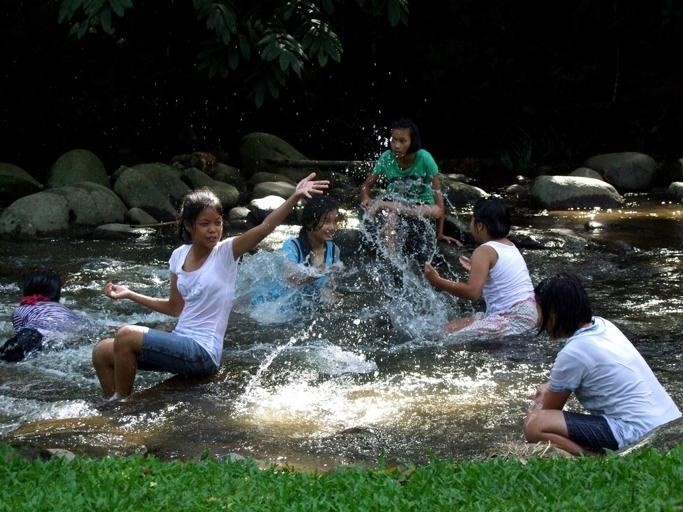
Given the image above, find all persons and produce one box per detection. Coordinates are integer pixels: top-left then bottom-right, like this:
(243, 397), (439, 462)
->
(250, 193), (343, 316)
(0, 269), (86, 362)
(356, 119), (465, 250)
(89, 171), (332, 405)
(420, 195), (542, 340)
(522, 269), (683, 458)
